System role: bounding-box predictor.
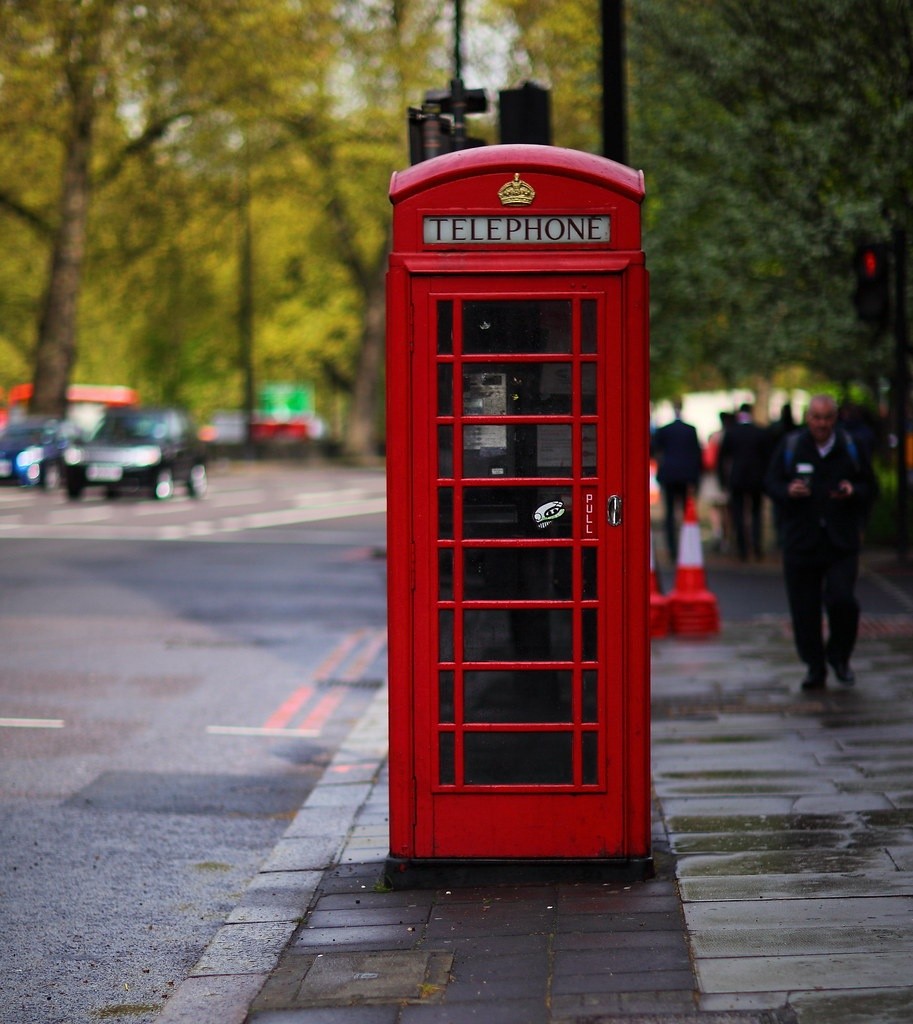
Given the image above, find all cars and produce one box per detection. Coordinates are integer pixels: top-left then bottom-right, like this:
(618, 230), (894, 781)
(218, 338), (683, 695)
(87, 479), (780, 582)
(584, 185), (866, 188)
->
(58, 407), (209, 500)
(0, 419), (90, 489)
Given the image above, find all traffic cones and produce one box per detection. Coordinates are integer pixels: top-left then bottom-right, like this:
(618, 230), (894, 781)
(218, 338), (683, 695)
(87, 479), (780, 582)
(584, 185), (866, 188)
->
(650, 492), (721, 640)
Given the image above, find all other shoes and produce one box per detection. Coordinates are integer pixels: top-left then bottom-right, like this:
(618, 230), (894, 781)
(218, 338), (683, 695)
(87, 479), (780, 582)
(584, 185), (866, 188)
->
(802, 669), (828, 690)
(826, 653), (855, 686)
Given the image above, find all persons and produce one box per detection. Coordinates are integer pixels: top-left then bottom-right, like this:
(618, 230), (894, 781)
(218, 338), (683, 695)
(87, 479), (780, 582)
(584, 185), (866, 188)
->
(702, 410), (735, 556)
(769, 393), (873, 691)
(836, 402), (864, 460)
(649, 394), (703, 564)
(761, 403), (799, 557)
(714, 402), (772, 567)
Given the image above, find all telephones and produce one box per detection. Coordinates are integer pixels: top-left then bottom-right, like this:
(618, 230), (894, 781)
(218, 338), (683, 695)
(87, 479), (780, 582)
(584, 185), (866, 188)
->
(438, 373), (517, 522)
